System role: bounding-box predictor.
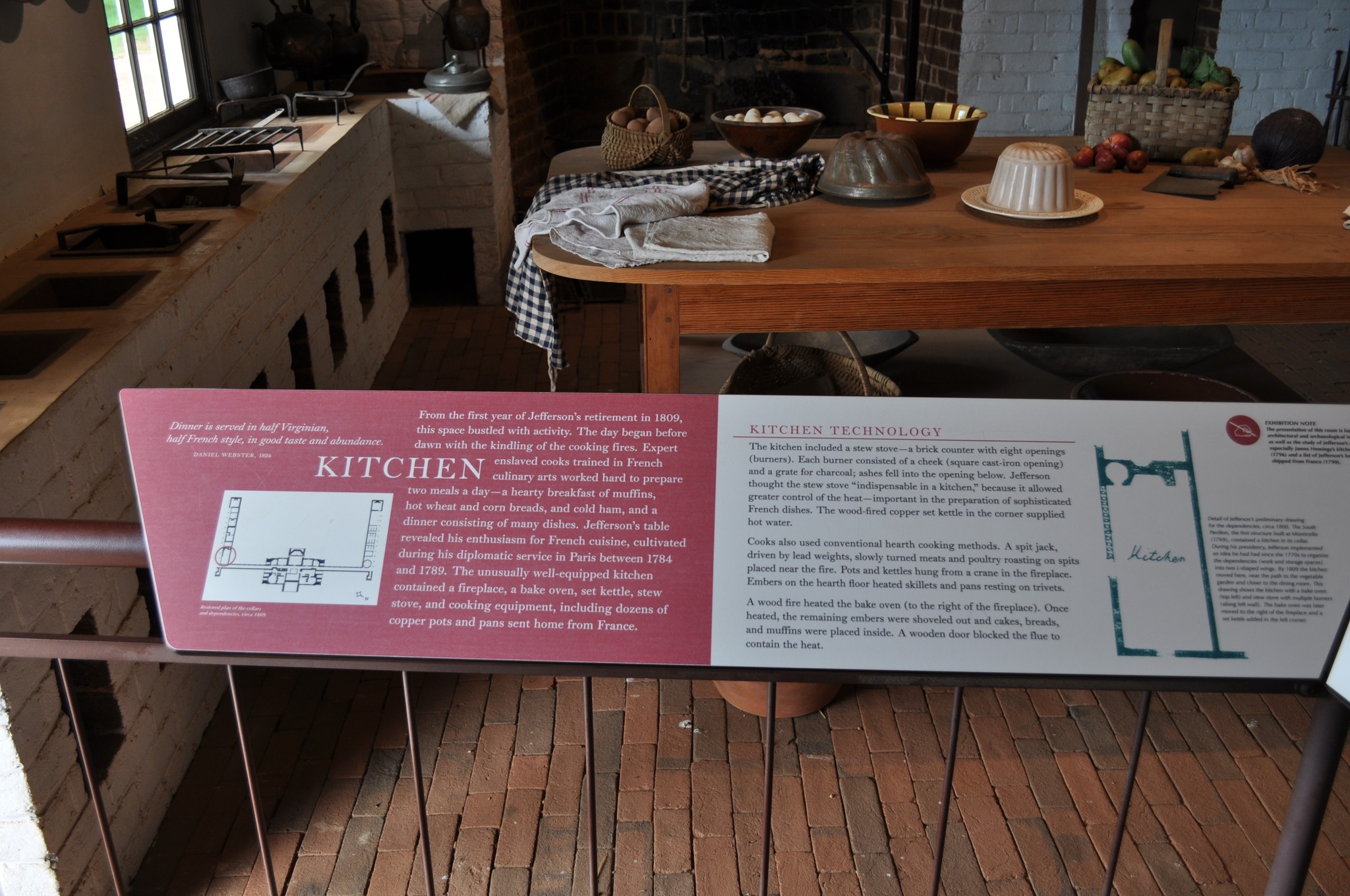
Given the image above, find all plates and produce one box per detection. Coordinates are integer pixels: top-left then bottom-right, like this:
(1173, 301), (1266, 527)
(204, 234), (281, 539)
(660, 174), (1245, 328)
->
(961, 182), (1104, 218)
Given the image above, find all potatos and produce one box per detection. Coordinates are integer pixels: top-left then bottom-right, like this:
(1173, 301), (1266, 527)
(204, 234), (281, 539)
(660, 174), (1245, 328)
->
(1098, 63), (1225, 89)
(1183, 146), (1223, 167)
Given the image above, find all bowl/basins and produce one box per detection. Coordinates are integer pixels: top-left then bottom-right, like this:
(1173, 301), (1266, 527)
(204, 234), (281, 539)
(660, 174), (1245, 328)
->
(711, 106), (825, 158)
(986, 141), (1075, 213)
(867, 102), (986, 167)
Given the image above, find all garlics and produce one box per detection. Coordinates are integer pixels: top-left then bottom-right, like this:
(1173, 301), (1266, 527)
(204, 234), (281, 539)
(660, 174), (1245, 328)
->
(1219, 143), (1254, 186)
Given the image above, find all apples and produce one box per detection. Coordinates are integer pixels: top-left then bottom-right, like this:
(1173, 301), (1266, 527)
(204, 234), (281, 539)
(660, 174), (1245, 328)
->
(1071, 132), (1149, 172)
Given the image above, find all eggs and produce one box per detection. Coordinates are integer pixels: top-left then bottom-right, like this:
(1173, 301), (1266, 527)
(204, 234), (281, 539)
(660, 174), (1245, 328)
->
(611, 106), (677, 133)
(725, 108), (813, 123)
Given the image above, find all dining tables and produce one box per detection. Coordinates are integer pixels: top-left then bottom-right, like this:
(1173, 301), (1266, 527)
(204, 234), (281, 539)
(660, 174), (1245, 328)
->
(531, 136), (1350, 395)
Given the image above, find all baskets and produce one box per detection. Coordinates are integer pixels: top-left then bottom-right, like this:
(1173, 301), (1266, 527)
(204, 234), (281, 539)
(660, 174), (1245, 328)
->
(719, 331), (902, 397)
(600, 83), (694, 170)
(1084, 18), (1240, 164)
